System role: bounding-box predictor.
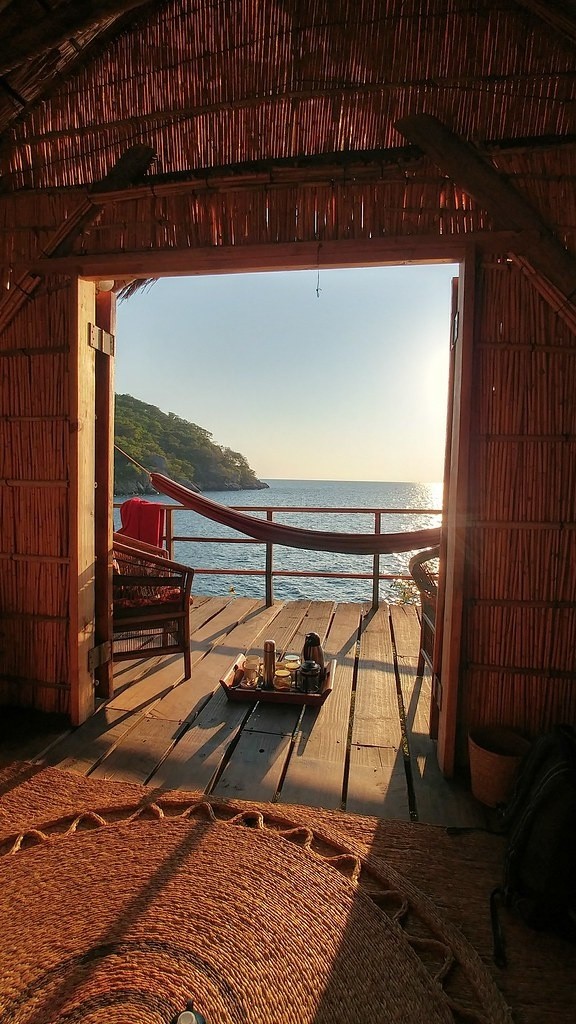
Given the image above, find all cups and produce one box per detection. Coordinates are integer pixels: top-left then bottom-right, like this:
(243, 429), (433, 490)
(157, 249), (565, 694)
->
(243, 655), (261, 674)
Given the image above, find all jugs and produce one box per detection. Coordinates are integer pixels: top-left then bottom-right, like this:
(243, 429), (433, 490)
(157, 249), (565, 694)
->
(300, 632), (326, 679)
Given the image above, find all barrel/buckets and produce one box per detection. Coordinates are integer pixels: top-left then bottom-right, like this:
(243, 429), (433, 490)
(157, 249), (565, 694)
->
(468, 729), (530, 807)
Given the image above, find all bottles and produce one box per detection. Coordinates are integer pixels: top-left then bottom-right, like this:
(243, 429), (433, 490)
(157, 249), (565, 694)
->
(273, 655), (320, 693)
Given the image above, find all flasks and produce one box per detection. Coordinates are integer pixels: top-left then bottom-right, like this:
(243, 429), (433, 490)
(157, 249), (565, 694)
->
(263, 639), (277, 689)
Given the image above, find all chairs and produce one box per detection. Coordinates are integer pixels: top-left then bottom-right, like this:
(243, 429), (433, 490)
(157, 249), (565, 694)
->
(112, 530), (195, 680)
(408, 546), (442, 677)
